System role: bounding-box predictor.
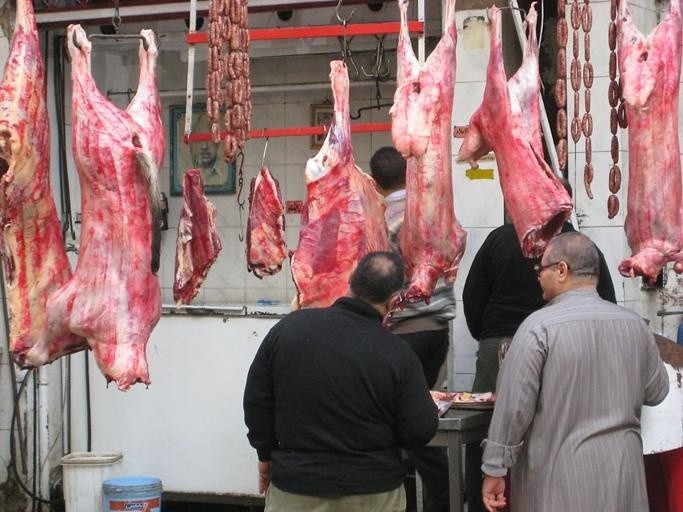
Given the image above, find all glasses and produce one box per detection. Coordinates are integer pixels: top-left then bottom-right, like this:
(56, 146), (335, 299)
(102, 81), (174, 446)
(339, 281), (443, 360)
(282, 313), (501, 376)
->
(533, 262), (571, 278)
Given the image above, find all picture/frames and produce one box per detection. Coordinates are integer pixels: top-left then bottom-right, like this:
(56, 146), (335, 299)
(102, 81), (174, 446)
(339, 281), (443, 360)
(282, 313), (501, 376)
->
(310, 104), (337, 150)
(170, 104), (236, 196)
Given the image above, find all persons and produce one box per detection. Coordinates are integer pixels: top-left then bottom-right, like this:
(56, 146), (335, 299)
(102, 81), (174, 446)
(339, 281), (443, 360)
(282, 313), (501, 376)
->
(359, 146), (456, 391)
(480, 231), (669, 511)
(244, 252), (438, 512)
(463, 181), (617, 512)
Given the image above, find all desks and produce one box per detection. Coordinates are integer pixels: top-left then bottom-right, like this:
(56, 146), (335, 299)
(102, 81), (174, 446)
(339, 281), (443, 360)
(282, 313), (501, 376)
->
(422, 405), (500, 512)
